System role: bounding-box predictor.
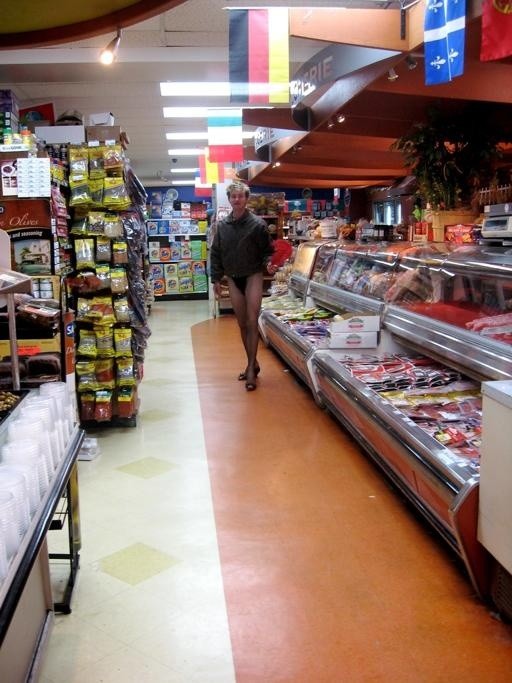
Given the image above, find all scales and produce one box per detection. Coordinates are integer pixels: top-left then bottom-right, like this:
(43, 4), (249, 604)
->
(478, 202), (512, 246)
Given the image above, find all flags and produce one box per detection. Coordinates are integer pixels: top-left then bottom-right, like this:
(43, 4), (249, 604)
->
(422, 0), (467, 86)
(478, 0), (511, 62)
(207, 107), (244, 163)
(199, 153), (225, 184)
(228, 7), (290, 106)
(194, 168), (212, 198)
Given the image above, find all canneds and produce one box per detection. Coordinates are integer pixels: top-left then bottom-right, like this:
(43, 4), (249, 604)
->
(373, 225), (394, 242)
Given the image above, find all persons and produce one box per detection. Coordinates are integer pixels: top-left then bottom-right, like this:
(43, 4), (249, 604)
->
(208, 179), (281, 392)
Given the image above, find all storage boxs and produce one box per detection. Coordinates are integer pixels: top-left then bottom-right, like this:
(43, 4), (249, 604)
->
(55, 121), (83, 125)
(34, 125), (85, 145)
(88, 111), (114, 126)
(84, 124), (121, 143)
(25, 120), (49, 133)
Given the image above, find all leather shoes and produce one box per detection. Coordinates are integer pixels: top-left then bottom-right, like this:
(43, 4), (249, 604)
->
(236, 364), (261, 391)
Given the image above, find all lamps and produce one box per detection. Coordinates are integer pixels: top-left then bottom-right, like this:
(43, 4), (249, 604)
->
(98, 29), (123, 65)
(272, 52), (418, 169)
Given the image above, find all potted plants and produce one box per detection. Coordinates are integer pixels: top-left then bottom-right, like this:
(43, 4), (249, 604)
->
(387, 120), (512, 243)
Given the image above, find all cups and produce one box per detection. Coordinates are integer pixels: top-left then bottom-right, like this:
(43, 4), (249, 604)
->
(0, 380), (78, 588)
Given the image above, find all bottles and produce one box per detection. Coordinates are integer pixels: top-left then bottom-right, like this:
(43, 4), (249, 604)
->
(414, 222), (434, 242)
(2, 127), (69, 165)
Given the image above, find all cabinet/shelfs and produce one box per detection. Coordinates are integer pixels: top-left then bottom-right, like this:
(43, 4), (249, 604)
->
(0, 140), (147, 433)
(256, 234), (511, 605)
(146, 217), (209, 296)
(0, 264), (85, 683)
(207, 204), (283, 320)
